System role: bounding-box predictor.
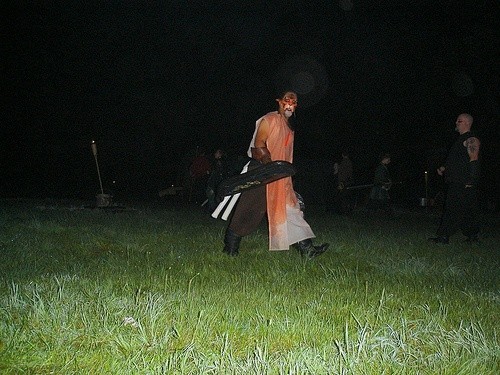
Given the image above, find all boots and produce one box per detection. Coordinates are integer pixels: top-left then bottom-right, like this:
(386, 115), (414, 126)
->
(222, 230), (241, 256)
(291, 238), (330, 261)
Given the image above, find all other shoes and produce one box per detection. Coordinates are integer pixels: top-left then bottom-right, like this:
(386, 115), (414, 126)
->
(463, 233), (480, 243)
(428, 234), (449, 245)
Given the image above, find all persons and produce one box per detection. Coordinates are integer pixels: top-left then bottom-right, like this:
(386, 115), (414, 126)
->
(369, 153), (400, 221)
(428, 113), (481, 245)
(211, 89), (329, 261)
(189, 145), (211, 207)
